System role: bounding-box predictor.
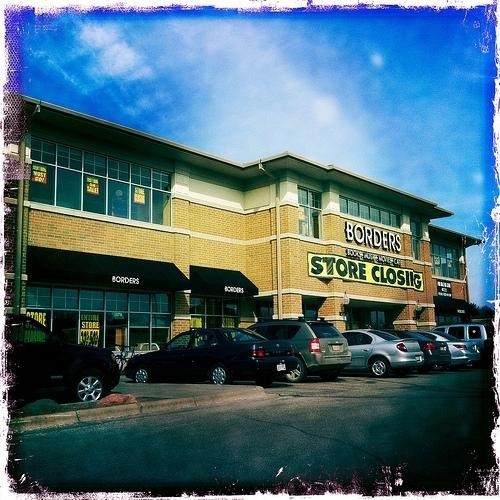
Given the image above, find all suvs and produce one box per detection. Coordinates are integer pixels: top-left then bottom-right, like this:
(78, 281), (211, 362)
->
(232, 315), (353, 384)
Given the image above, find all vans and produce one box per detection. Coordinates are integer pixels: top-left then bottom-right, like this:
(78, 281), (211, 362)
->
(429, 321), (493, 357)
(133, 340), (161, 350)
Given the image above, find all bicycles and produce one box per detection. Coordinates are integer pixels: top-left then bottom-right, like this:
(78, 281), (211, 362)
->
(111, 343), (137, 375)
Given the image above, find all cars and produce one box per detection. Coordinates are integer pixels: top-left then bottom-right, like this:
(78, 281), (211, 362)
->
(7, 311), (124, 405)
(336, 324), (426, 379)
(382, 326), (452, 374)
(413, 328), (482, 372)
(123, 326), (299, 387)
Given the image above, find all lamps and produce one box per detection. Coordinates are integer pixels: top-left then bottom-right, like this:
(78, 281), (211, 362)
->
(415, 299), (422, 312)
(342, 291), (351, 305)
